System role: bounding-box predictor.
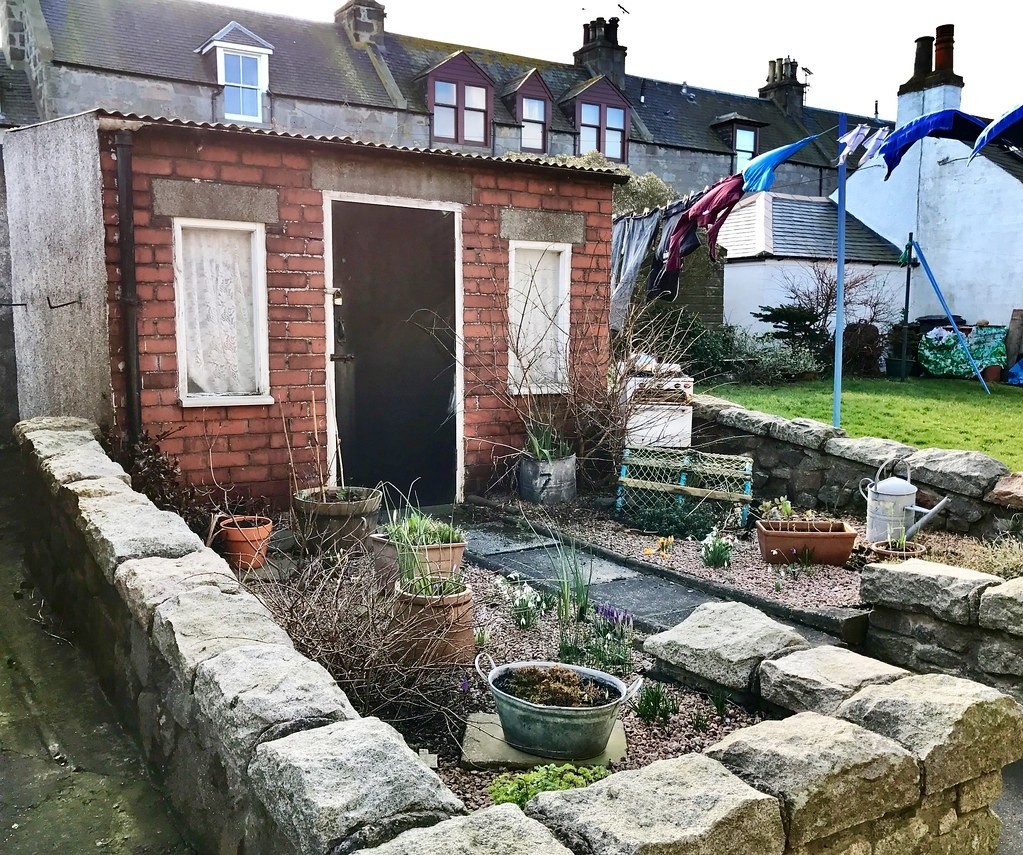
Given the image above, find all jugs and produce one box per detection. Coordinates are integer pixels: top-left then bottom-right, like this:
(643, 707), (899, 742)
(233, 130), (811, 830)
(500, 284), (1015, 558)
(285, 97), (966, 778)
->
(858, 458), (951, 540)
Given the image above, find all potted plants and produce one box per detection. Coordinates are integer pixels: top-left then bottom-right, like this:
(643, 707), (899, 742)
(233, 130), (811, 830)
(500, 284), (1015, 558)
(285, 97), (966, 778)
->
(870, 502), (927, 562)
(291, 485), (383, 559)
(519, 409), (577, 505)
(388, 513), (477, 669)
(369, 510), (468, 597)
(884, 353), (916, 378)
(755, 494), (858, 569)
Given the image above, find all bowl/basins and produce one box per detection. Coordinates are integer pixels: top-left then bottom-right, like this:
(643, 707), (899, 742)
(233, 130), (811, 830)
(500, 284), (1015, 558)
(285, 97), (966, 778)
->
(884, 357), (916, 378)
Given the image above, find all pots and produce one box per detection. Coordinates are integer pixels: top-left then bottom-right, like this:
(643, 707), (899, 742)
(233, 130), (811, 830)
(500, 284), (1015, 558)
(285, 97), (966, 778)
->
(475, 653), (643, 760)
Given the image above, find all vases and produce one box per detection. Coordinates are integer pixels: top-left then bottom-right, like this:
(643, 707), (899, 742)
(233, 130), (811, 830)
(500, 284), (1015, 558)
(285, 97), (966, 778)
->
(985, 365), (1001, 382)
(219, 516), (271, 570)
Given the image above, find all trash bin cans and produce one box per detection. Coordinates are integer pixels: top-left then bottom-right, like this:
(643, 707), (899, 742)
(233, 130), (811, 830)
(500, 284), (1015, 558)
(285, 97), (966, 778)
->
(916, 315), (967, 378)
